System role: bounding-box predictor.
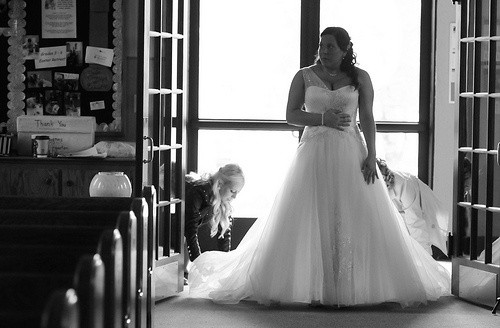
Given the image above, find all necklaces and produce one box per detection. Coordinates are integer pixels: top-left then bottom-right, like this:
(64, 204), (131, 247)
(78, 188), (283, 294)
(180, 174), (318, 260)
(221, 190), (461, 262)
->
(323, 68), (343, 77)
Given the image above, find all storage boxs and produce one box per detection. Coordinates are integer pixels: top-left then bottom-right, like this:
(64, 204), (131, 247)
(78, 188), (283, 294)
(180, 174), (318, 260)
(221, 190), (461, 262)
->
(16, 115), (96, 157)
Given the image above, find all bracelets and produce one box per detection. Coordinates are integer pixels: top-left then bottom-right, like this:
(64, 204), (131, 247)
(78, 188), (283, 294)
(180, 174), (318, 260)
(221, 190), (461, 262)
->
(321, 111), (324, 127)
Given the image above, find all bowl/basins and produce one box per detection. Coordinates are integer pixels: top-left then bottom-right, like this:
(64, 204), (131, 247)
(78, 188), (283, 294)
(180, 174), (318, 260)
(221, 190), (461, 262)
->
(89, 172), (132, 197)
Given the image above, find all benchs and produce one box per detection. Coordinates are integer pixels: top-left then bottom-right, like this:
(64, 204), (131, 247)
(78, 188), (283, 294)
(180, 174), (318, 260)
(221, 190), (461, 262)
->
(0, 185), (158, 328)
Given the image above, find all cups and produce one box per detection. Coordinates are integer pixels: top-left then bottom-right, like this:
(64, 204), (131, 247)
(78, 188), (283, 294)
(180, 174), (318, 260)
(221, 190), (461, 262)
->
(31, 135), (38, 156)
(35, 136), (49, 158)
(49, 138), (63, 157)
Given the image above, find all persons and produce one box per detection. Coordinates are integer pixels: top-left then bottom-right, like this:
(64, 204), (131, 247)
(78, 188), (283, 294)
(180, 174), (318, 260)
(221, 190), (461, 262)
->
(188, 28), (453, 309)
(184, 163), (246, 286)
(373, 157), (454, 262)
